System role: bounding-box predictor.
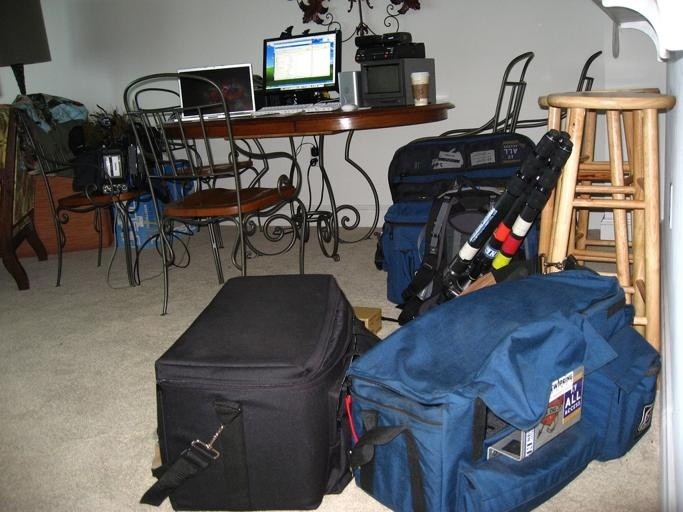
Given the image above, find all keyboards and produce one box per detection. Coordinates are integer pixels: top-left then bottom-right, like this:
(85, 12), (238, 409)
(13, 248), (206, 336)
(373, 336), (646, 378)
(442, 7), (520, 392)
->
(254, 102), (341, 118)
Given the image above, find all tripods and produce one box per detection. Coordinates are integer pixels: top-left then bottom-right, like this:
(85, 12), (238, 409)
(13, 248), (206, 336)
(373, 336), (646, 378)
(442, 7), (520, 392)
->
(428, 130), (574, 311)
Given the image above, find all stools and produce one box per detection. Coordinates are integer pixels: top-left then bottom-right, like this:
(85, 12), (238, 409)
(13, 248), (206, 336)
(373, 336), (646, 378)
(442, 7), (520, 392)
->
(536, 86), (675, 361)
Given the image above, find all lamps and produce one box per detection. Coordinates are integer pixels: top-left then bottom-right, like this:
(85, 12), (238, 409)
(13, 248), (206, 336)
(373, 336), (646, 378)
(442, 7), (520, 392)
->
(0, 0), (50, 98)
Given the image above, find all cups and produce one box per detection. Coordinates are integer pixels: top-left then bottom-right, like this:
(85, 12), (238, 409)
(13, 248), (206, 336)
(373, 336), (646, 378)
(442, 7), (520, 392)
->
(410, 71), (429, 105)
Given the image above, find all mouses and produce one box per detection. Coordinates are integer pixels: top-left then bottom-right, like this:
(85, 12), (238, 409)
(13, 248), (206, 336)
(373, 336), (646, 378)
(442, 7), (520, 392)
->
(342, 104), (357, 112)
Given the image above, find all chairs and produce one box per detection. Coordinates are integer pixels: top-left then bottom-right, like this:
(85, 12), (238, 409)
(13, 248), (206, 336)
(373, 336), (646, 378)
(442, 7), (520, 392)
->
(440, 48), (600, 137)
(3, 99), (50, 290)
(124, 72), (306, 314)
(22, 106), (159, 287)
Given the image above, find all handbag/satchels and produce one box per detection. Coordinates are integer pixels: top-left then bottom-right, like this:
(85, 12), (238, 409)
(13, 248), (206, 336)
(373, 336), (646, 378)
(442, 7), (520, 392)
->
(139, 275), (381, 511)
(380, 135), (541, 303)
(349, 267), (661, 512)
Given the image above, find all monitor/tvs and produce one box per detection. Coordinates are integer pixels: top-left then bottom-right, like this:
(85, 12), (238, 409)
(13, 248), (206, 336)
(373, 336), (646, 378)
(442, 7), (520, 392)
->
(360, 58), (436, 107)
(263, 30), (342, 104)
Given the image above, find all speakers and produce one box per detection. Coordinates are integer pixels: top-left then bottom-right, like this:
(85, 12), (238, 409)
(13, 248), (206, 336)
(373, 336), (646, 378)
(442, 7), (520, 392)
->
(338, 72), (362, 107)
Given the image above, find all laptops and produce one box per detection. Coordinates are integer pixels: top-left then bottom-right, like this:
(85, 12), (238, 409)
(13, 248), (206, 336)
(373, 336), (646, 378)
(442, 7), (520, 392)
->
(173, 63), (256, 122)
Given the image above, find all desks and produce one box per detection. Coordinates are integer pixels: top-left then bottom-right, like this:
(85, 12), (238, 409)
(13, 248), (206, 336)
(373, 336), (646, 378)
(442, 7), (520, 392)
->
(112, 104), (454, 267)
